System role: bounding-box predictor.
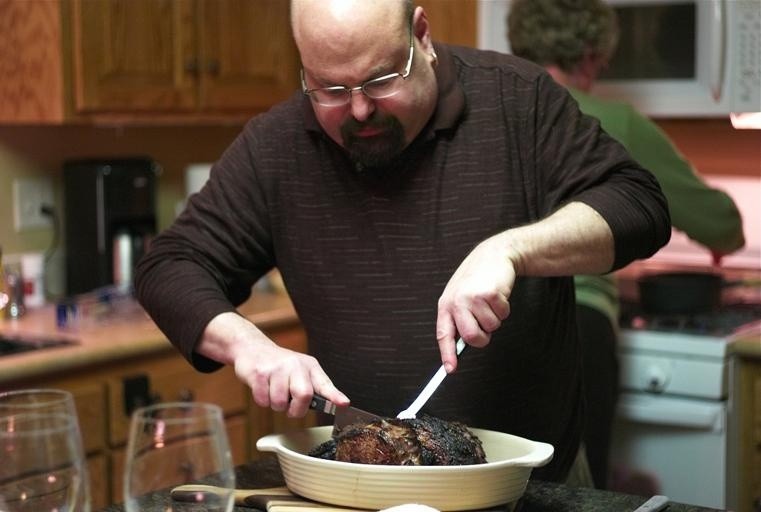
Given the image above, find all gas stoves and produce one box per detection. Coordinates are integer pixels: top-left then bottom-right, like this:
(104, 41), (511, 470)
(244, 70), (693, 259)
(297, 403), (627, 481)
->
(615, 296), (761, 336)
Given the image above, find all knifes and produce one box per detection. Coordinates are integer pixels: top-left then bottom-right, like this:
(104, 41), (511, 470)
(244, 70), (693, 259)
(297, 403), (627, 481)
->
(285, 391), (389, 439)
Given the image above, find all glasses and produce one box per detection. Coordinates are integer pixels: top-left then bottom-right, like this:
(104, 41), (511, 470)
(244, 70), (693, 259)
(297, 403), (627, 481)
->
(301, 8), (414, 108)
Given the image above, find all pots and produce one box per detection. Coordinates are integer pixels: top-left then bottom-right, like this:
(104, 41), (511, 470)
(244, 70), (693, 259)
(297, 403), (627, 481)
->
(634, 269), (742, 311)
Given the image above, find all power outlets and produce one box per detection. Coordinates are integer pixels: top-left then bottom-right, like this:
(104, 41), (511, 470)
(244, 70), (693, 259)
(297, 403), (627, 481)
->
(13, 176), (55, 235)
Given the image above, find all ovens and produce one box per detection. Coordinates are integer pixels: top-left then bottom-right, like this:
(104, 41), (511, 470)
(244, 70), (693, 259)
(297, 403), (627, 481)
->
(604, 334), (727, 511)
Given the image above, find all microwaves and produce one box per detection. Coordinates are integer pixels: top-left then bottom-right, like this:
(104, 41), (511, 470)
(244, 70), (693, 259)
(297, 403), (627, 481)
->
(475, 0), (761, 117)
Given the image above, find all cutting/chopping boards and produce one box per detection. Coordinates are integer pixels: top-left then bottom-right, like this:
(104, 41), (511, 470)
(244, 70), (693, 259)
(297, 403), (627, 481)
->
(167, 483), (378, 512)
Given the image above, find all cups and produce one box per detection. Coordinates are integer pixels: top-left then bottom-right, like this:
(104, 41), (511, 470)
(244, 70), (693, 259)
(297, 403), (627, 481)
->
(120, 401), (238, 512)
(0, 390), (90, 512)
(0, 414), (82, 512)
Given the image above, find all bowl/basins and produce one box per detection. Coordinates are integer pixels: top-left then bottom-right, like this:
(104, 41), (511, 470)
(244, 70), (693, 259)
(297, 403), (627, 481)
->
(255, 425), (555, 511)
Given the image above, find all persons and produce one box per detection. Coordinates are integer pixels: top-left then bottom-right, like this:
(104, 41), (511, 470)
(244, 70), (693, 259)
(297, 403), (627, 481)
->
(507, 0), (747, 491)
(132, 0), (672, 491)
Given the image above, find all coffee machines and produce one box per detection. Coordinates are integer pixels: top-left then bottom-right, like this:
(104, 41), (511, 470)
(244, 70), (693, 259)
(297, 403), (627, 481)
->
(60, 152), (161, 313)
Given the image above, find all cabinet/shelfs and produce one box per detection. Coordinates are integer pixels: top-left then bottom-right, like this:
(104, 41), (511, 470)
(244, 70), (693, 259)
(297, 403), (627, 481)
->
(3, 380), (109, 512)
(108, 353), (251, 504)
(1, 0), (305, 128)
(413, 1), (480, 53)
(249, 324), (312, 467)
